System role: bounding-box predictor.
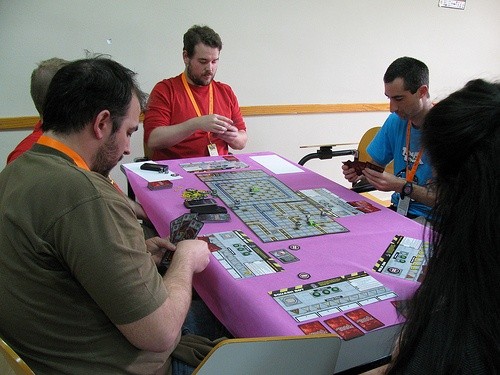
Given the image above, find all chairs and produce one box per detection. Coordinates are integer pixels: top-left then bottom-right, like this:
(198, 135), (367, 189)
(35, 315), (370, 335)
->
(297, 126), (397, 207)
(191, 334), (341, 375)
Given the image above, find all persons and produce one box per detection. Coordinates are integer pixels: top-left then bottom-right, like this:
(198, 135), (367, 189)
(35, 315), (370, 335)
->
(143, 25), (248, 162)
(7, 56), (74, 174)
(364, 78), (500, 375)
(0, 55), (233, 375)
(342, 56), (442, 228)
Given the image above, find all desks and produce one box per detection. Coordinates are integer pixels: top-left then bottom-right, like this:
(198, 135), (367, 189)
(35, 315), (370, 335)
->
(120, 152), (444, 375)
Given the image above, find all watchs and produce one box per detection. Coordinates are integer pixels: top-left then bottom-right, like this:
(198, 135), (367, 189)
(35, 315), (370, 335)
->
(400, 182), (412, 200)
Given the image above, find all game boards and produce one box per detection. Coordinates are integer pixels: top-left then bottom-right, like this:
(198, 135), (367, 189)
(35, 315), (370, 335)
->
(196, 169), (352, 244)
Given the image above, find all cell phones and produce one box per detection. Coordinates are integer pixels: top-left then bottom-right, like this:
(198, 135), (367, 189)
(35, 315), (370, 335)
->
(197, 214), (231, 223)
(184, 198), (217, 209)
(141, 163), (168, 172)
(191, 205), (227, 214)
